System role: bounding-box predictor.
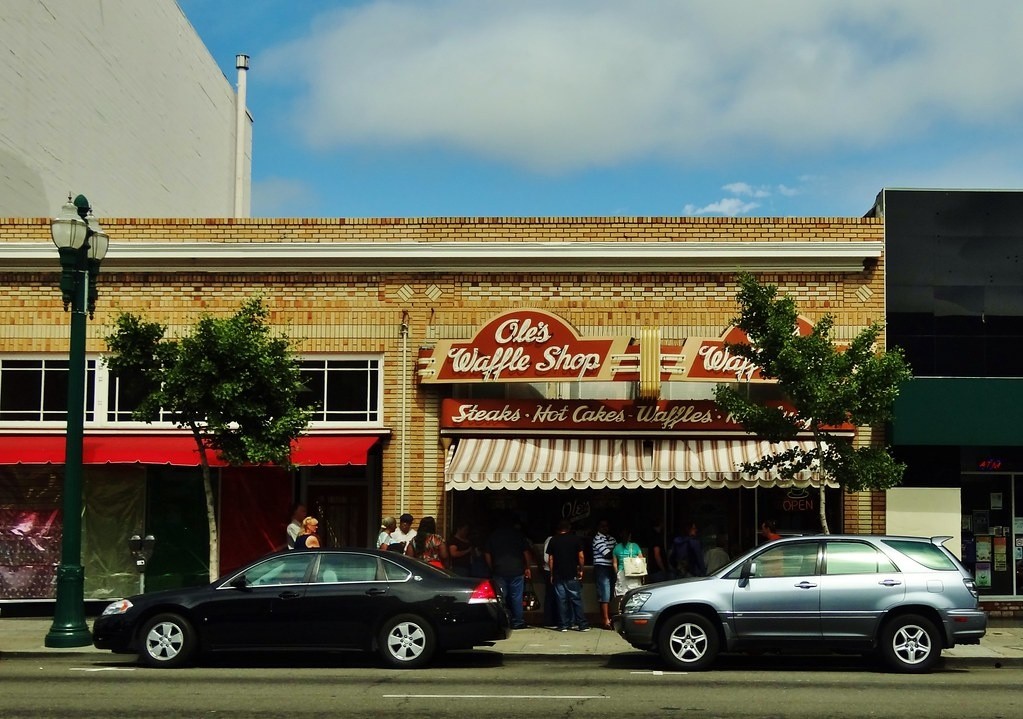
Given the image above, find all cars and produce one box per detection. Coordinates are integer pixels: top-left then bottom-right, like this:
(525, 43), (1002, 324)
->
(92, 548), (514, 670)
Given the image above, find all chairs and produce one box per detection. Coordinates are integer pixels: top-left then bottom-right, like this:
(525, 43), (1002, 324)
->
(322, 570), (338, 582)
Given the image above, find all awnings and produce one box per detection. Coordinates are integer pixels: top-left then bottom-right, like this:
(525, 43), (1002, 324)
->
(884, 379), (1023, 473)
(0, 434), (379, 465)
(444, 436), (842, 491)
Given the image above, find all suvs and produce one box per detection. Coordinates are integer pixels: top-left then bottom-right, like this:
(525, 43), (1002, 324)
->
(612, 534), (988, 672)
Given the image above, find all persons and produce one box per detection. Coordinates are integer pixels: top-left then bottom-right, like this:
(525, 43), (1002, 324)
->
(295, 516), (320, 548)
(589, 520), (619, 628)
(447, 519), (478, 578)
(375, 514), (450, 571)
(482, 513), (534, 629)
(539, 516), (593, 630)
(285, 504), (306, 549)
(611, 528), (649, 607)
(646, 518), (730, 583)
(762, 519), (782, 543)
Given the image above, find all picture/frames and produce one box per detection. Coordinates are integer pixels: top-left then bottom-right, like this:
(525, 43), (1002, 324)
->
(962, 515), (972, 532)
(972, 510), (989, 534)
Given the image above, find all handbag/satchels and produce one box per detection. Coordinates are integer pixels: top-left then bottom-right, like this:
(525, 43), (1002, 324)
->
(522, 575), (541, 612)
(623, 542), (648, 576)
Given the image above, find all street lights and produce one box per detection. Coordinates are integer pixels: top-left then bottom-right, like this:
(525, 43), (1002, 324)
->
(41, 190), (110, 648)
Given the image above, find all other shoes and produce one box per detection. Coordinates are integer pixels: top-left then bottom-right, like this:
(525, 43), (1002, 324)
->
(562, 629), (567, 632)
(515, 622), (529, 629)
(602, 623), (613, 630)
(581, 627), (591, 632)
